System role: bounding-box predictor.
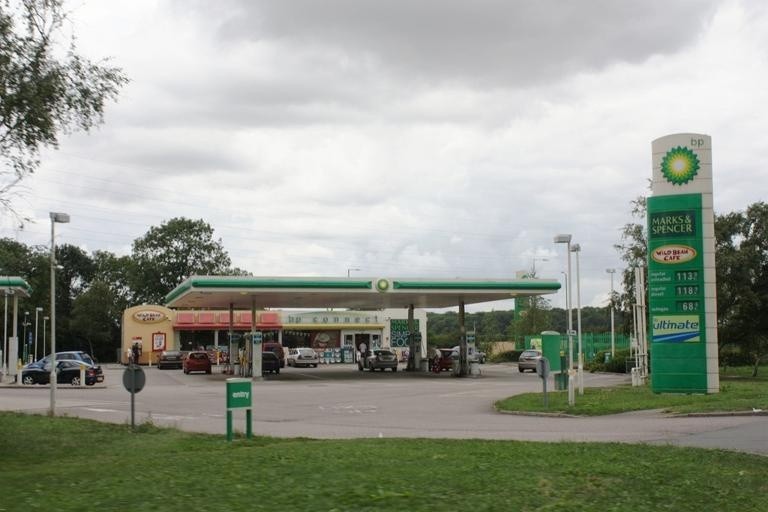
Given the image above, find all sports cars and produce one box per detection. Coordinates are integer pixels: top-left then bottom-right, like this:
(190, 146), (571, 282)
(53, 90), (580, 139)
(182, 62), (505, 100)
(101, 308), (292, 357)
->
(13, 359), (105, 386)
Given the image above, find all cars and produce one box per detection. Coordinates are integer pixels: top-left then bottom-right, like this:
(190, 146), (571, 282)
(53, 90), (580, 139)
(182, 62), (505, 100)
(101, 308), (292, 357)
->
(155, 350), (184, 369)
(356, 350), (398, 372)
(434, 349), (460, 371)
(452, 345), (487, 365)
(182, 352), (213, 374)
(24, 351), (94, 368)
(287, 348), (319, 369)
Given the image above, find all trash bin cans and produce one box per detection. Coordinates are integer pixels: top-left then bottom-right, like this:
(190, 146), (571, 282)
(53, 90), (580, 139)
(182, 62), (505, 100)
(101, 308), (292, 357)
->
(554, 373), (569, 390)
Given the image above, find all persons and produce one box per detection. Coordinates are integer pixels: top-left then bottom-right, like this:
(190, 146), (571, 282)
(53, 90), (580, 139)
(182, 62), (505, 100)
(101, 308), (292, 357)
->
(127, 342), (139, 365)
(358, 341), (368, 358)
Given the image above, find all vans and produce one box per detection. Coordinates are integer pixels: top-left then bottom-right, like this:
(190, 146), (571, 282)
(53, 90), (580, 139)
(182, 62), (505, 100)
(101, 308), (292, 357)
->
(263, 343), (286, 367)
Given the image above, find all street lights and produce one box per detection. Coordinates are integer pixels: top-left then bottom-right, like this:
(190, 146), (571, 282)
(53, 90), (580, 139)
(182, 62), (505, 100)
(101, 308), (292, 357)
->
(603, 267), (617, 359)
(551, 231), (586, 404)
(49, 211), (74, 420)
(530, 255), (547, 329)
(18, 306), (48, 366)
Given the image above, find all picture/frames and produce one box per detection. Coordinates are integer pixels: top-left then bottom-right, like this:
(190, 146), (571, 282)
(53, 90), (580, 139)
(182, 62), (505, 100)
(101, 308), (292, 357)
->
(152, 332), (167, 352)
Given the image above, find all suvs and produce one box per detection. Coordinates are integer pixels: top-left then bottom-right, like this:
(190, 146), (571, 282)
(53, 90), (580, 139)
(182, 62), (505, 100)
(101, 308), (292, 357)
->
(260, 351), (280, 373)
(517, 349), (542, 373)
